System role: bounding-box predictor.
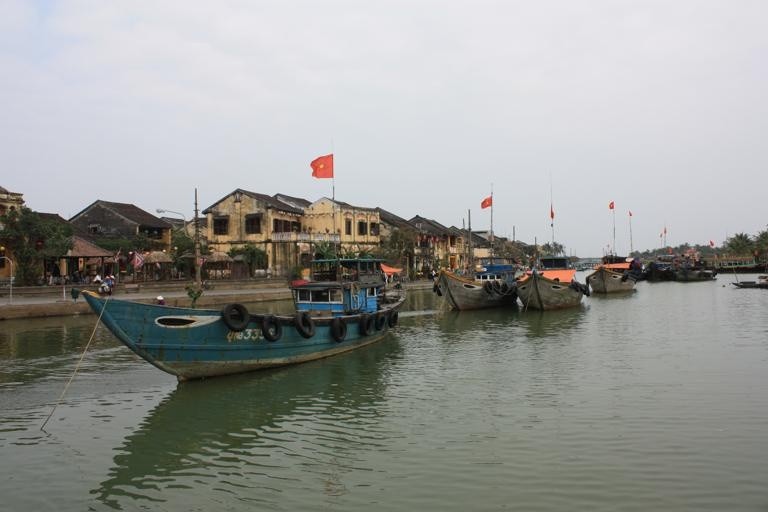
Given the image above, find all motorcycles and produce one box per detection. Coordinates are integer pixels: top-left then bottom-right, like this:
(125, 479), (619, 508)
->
(97, 282), (113, 295)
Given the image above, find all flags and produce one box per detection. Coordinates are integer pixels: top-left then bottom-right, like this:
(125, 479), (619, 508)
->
(380, 263), (404, 276)
(629, 210), (632, 216)
(480, 195), (492, 209)
(311, 154), (333, 179)
(710, 239), (714, 246)
(664, 226), (667, 233)
(609, 201), (615, 210)
(550, 205), (554, 219)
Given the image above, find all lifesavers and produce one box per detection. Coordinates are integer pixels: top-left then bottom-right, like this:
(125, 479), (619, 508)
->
(290, 279), (310, 288)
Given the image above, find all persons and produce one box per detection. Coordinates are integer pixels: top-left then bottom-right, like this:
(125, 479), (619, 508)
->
(92, 272), (116, 295)
(157, 295), (167, 306)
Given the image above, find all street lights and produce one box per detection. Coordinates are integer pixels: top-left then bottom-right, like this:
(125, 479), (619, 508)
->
(154, 204), (193, 240)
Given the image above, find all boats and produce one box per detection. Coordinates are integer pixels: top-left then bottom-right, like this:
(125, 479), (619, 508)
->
(72, 241), (407, 385)
(432, 303), (588, 338)
(95, 330), (403, 493)
